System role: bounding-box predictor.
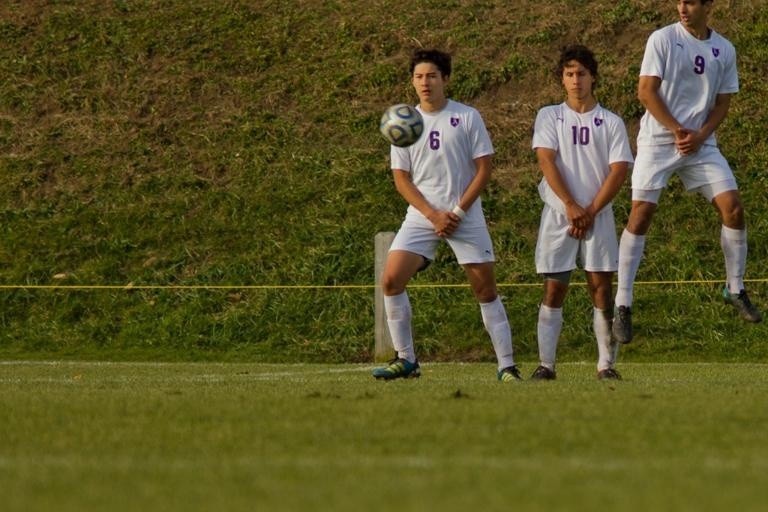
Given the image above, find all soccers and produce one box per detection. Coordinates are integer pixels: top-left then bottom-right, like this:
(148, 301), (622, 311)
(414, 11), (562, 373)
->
(380, 104), (423, 147)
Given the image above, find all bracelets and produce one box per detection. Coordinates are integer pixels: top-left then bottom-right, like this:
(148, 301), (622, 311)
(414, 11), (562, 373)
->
(451, 204), (466, 221)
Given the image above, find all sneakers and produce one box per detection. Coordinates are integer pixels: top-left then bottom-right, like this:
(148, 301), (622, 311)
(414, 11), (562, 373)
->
(722, 285), (761, 323)
(497, 365), (523, 382)
(373, 353), (420, 380)
(529, 365), (556, 381)
(611, 298), (633, 344)
(597, 368), (623, 381)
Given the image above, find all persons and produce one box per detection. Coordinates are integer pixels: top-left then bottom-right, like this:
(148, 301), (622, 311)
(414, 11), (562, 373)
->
(372, 48), (524, 384)
(530, 46), (634, 381)
(610, 0), (763, 344)
